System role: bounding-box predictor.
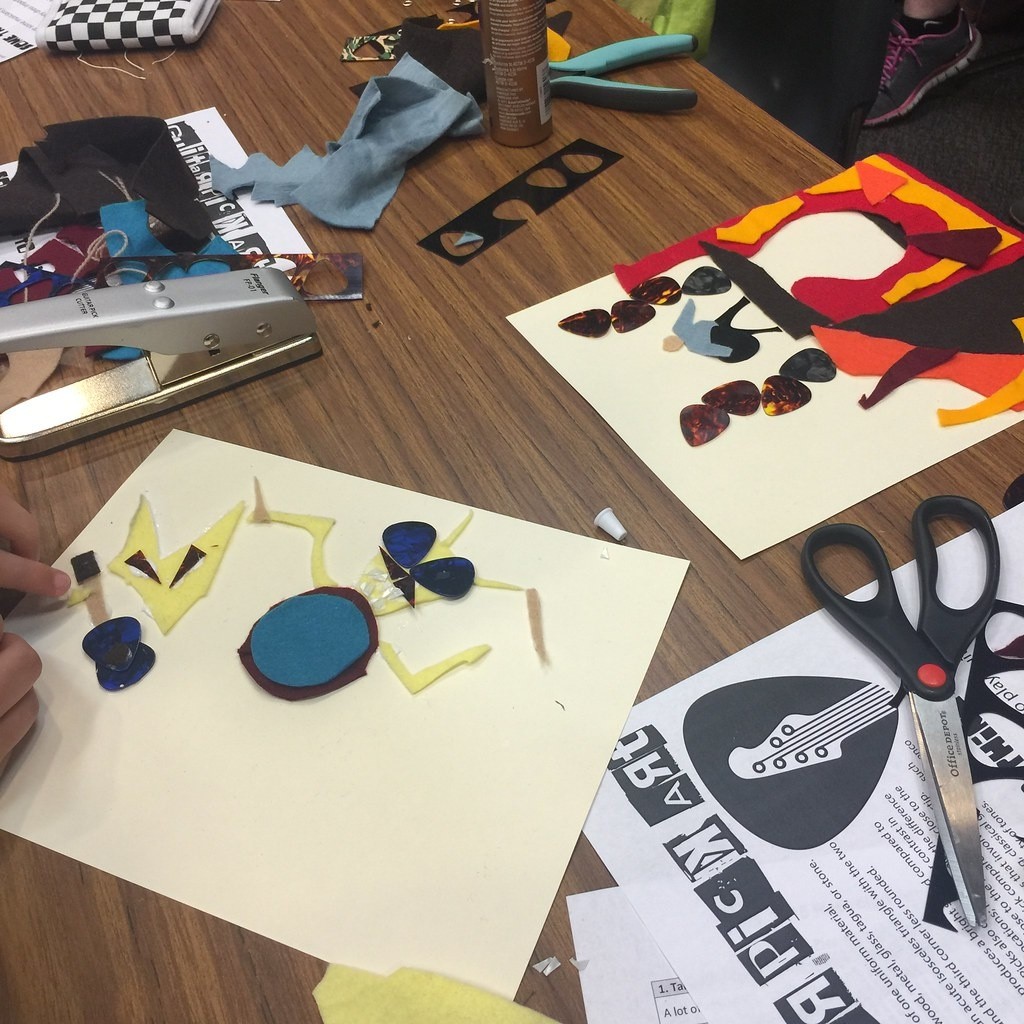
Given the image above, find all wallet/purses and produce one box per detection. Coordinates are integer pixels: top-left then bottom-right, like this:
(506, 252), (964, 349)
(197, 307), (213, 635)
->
(44, 0), (220, 52)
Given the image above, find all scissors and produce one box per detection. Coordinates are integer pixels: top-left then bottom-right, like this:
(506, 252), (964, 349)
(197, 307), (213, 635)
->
(801, 496), (1000, 926)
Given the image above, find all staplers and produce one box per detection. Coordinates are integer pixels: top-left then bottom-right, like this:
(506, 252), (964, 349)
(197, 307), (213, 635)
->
(0, 268), (325, 463)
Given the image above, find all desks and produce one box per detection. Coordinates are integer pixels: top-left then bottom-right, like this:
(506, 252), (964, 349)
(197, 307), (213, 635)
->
(0, 0), (1024, 1024)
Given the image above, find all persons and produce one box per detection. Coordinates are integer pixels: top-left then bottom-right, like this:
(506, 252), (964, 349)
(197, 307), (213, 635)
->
(0, 490), (72, 780)
(1009, 199), (1024, 226)
(864, 0), (982, 126)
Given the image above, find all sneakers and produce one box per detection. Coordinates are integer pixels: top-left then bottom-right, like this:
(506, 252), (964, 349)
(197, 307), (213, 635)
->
(862, 9), (983, 126)
